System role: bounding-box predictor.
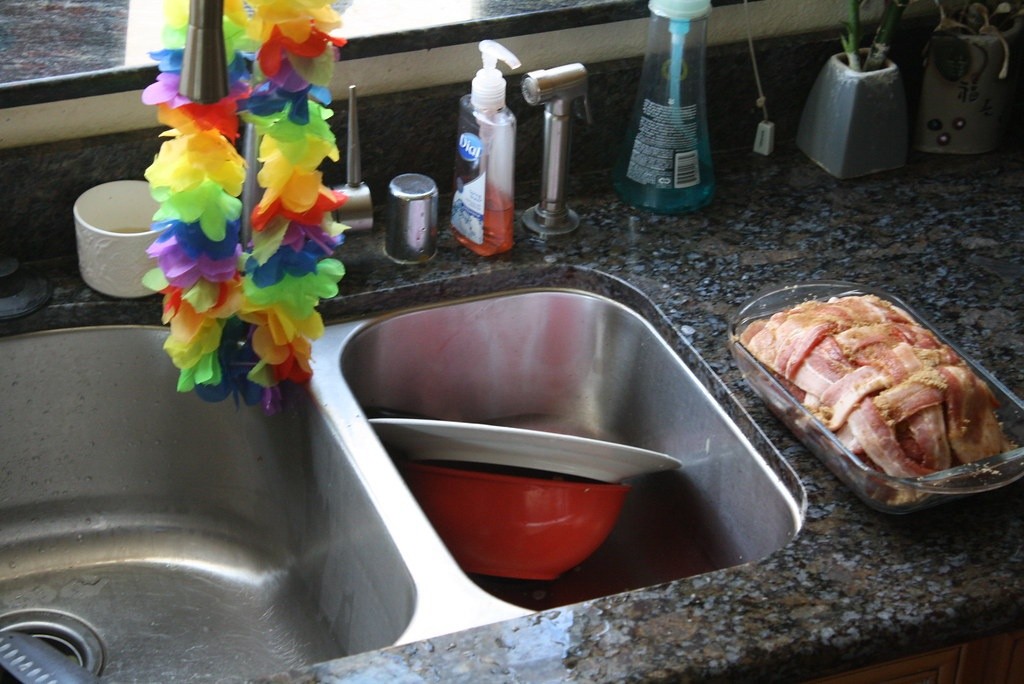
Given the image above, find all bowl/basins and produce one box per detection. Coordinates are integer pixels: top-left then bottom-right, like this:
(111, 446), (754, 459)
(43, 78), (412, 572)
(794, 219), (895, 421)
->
(728, 280), (1024, 513)
(396, 461), (633, 580)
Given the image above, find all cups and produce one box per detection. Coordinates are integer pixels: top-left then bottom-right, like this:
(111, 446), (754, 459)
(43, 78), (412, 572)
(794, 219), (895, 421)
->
(916, 12), (1024, 152)
(73, 180), (161, 297)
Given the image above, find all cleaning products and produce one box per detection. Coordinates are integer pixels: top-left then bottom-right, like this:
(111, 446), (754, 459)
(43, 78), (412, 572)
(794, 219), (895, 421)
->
(609, 2), (715, 215)
(449, 41), (521, 256)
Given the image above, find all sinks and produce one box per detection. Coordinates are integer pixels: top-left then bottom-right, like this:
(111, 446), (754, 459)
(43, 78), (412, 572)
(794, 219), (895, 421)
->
(0, 326), (414, 684)
(340, 289), (802, 622)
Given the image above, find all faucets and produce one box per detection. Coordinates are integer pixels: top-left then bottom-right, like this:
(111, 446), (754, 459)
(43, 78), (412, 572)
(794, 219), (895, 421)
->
(176, 0), (374, 255)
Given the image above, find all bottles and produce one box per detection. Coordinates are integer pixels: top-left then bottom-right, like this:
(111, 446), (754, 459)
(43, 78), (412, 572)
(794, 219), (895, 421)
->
(613, 0), (717, 213)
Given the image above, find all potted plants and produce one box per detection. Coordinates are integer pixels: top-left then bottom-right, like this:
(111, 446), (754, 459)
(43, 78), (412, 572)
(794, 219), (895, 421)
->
(792, 0), (914, 180)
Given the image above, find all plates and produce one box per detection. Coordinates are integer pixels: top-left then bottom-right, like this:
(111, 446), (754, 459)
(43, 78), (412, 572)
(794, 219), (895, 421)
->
(369, 417), (684, 483)
